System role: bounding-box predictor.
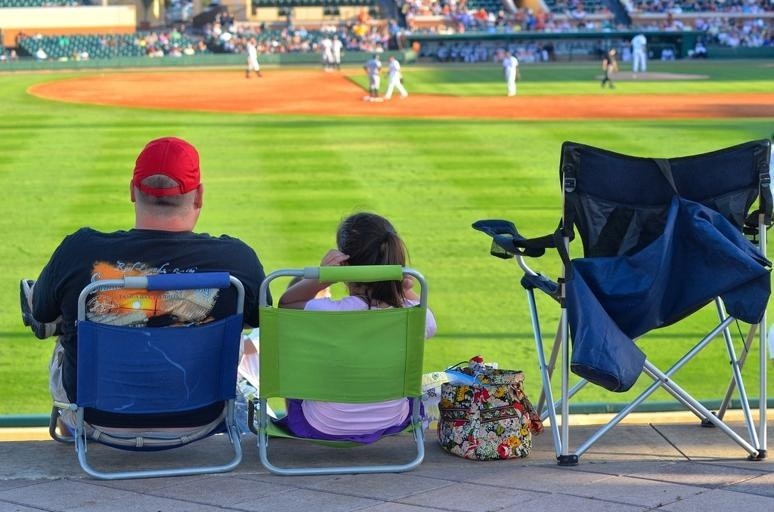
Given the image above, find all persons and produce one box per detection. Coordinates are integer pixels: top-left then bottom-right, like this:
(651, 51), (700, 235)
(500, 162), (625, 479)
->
(258, 26), (322, 53)
(601, 48), (618, 89)
(245, 37), (263, 79)
(245, 21), (261, 39)
(632, 0), (647, 35)
(320, 34), (336, 71)
(265, 212), (437, 451)
(501, 49), (521, 97)
(346, 0), (504, 63)
(363, 53), (384, 101)
(0, 8), (245, 62)
(331, 35), (343, 70)
(632, 31), (646, 72)
(517, 0), (630, 62)
(647, 0), (774, 59)
(19, 136), (275, 452)
(386, 55), (409, 100)
(504, 1), (517, 54)
(322, 23), (344, 39)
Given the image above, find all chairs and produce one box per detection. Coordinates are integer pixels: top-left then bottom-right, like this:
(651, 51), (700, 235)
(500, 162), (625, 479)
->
(20, 279), (63, 339)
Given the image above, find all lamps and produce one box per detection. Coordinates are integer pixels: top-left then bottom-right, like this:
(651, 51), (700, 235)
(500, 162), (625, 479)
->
(20, 270), (245, 483)
(472, 139), (774, 466)
(245, 265), (430, 477)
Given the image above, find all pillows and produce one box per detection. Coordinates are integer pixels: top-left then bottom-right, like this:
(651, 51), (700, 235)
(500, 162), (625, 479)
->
(134, 139), (200, 196)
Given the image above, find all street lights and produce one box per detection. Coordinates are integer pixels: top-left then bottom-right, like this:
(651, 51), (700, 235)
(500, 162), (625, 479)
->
(437, 367), (543, 460)
